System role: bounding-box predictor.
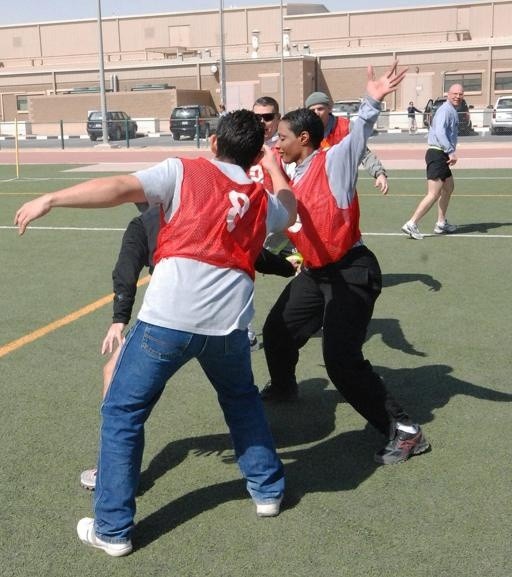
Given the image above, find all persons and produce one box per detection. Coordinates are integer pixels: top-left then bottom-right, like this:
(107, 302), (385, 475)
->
(407, 100), (423, 136)
(258, 59), (431, 466)
(305, 90), (389, 196)
(401, 84), (465, 239)
(79, 199), (301, 488)
(14, 110), (299, 557)
(245, 96), (297, 254)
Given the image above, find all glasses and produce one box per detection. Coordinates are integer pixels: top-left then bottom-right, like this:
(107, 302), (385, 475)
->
(255, 112), (278, 122)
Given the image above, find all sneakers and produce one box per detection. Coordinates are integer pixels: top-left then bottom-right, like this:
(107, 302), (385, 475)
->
(373, 417), (431, 466)
(74, 515), (131, 557)
(255, 488), (283, 517)
(434, 219), (458, 234)
(260, 381), (301, 406)
(401, 220), (424, 240)
(81, 466), (97, 491)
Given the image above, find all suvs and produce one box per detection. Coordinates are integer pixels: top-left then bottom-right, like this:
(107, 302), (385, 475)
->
(422, 96), (475, 133)
(86, 110), (138, 141)
(331, 100), (364, 119)
(168, 104), (221, 140)
(486, 94), (512, 135)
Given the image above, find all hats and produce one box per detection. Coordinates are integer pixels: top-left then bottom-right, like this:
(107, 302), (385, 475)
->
(304, 91), (330, 110)
